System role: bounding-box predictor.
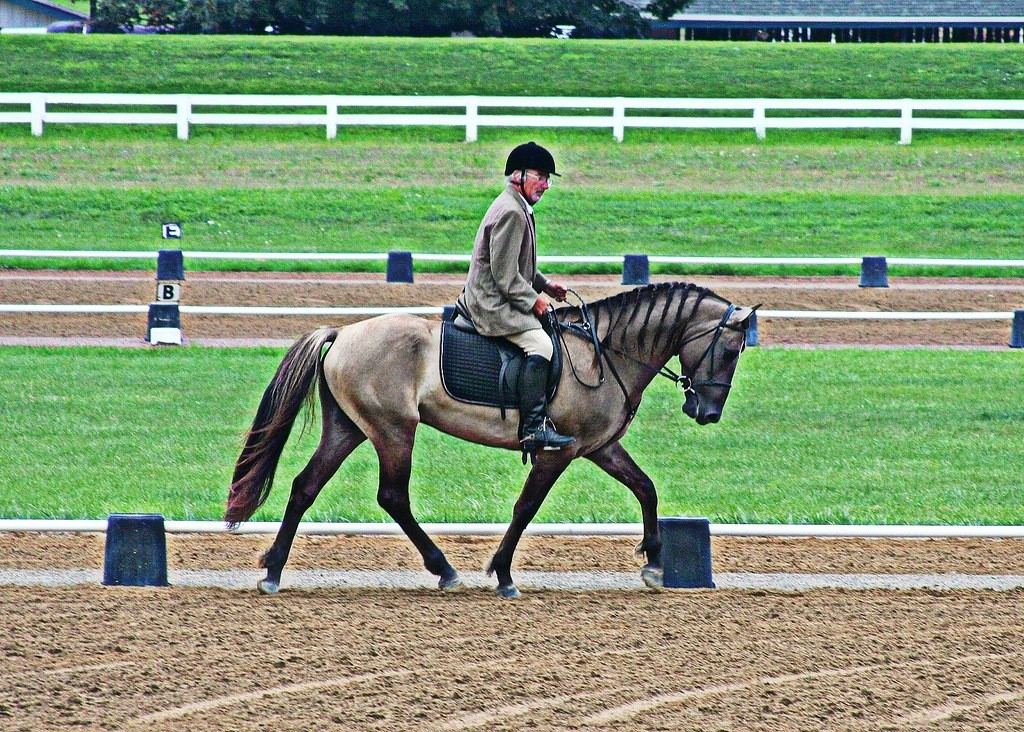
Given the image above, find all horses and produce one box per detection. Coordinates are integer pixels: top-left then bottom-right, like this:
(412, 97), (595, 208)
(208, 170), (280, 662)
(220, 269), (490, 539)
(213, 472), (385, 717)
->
(219, 281), (764, 598)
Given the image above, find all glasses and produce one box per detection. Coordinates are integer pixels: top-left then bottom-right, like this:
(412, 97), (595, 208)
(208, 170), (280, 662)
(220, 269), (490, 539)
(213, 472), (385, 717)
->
(525, 172), (555, 185)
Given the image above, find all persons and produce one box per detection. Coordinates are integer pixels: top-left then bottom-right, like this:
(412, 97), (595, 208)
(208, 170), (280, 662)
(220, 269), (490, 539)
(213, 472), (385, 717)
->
(457, 138), (580, 457)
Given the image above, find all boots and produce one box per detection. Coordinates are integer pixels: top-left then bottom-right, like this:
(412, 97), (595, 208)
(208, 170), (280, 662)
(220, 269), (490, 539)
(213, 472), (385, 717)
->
(517, 354), (576, 450)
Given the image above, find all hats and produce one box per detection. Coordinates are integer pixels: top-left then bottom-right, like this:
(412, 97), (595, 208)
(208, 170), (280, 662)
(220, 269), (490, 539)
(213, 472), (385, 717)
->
(504, 140), (562, 177)
